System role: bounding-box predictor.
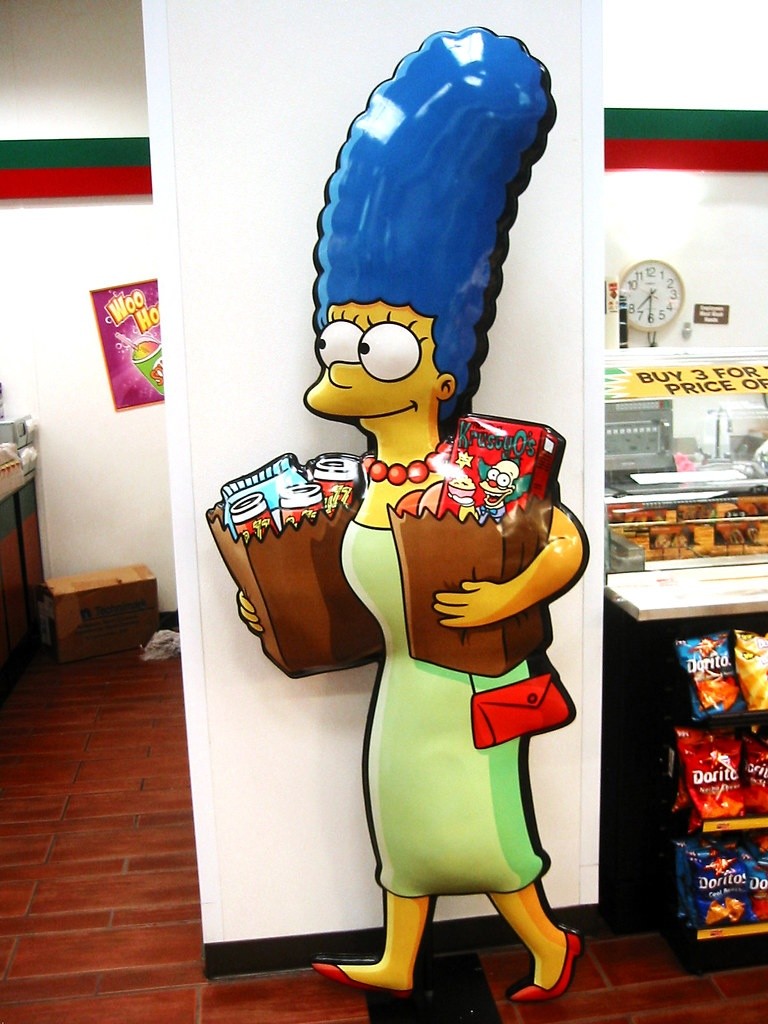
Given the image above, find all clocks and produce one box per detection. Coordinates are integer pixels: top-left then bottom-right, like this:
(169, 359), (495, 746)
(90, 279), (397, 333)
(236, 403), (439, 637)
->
(617, 259), (686, 331)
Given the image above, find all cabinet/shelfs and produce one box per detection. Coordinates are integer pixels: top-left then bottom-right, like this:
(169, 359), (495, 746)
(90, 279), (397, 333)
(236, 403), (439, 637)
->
(625, 613), (768, 974)
(0, 473), (45, 711)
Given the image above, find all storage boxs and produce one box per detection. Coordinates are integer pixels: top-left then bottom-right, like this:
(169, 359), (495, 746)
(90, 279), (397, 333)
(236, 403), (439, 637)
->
(31, 563), (160, 666)
